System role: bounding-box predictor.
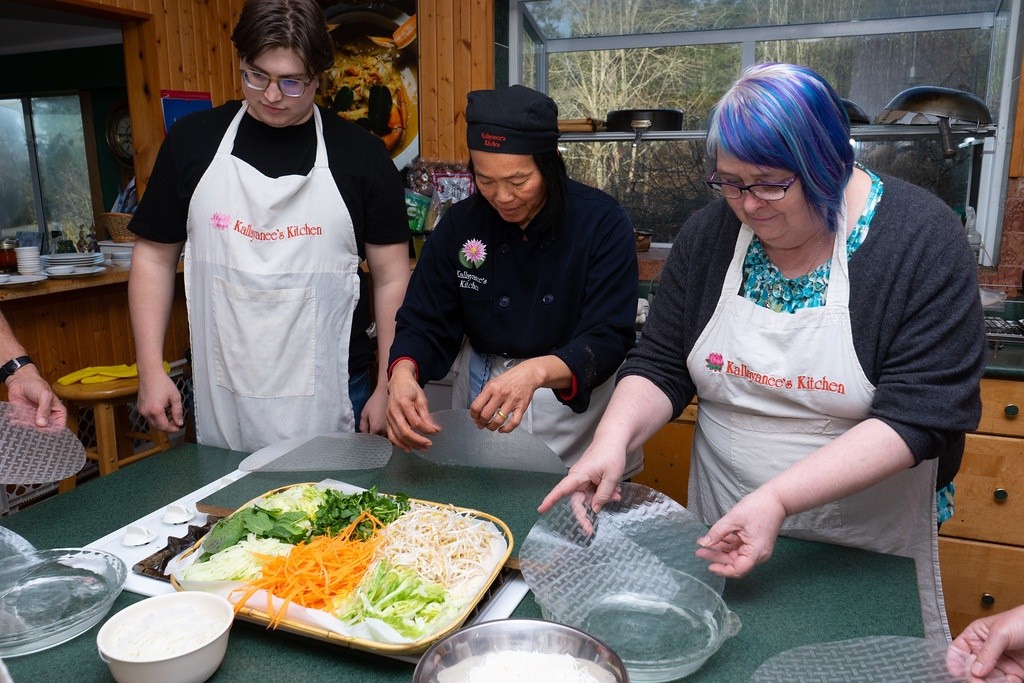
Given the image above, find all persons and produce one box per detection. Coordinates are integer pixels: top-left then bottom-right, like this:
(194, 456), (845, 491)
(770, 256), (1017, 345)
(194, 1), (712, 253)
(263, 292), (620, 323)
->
(537, 62), (989, 647)
(946, 603), (1024, 683)
(385, 86), (645, 482)
(127, 0), (411, 454)
(0, 310), (67, 433)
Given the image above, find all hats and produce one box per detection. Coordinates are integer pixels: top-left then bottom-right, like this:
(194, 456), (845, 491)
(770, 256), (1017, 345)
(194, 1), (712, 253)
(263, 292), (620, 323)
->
(464, 84), (563, 154)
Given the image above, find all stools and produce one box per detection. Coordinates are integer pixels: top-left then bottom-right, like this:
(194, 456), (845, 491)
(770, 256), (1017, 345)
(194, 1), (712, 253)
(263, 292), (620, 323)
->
(51, 376), (171, 494)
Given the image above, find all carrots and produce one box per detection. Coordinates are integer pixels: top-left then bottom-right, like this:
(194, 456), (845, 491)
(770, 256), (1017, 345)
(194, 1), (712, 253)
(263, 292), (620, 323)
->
(228, 510), (387, 630)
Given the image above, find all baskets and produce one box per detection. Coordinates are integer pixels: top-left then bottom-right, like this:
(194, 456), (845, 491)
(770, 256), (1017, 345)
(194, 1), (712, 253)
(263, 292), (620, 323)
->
(97, 210), (142, 242)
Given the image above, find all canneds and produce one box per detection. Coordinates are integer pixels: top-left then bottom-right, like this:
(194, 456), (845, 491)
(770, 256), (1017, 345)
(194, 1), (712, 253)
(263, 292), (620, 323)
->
(0, 244), (18, 272)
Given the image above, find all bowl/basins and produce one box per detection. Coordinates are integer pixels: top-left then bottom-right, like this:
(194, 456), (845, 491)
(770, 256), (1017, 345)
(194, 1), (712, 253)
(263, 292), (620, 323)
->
(112, 253), (131, 268)
(0, 547), (124, 660)
(412, 617), (628, 682)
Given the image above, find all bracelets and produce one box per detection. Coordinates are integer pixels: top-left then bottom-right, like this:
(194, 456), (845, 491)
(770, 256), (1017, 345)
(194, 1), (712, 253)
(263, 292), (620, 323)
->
(0, 356), (33, 383)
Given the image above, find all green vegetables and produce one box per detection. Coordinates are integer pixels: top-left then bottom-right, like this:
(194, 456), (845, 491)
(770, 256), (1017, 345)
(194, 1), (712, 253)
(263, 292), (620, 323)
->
(199, 503), (312, 563)
(333, 557), (448, 638)
(309, 486), (409, 542)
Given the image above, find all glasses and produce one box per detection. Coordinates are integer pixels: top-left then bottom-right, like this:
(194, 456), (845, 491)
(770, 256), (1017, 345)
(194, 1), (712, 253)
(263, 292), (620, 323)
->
(238, 59), (319, 97)
(706, 168), (800, 202)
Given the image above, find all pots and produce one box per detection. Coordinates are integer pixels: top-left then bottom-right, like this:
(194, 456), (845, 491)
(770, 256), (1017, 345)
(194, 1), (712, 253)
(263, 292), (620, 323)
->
(633, 227), (655, 252)
(838, 98), (871, 124)
(872, 109), (984, 158)
(884, 86), (993, 133)
(0, 240), (17, 270)
(606, 108), (684, 150)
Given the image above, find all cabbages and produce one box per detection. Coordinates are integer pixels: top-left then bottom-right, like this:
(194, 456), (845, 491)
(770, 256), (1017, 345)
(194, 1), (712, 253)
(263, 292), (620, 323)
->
(264, 484), (328, 519)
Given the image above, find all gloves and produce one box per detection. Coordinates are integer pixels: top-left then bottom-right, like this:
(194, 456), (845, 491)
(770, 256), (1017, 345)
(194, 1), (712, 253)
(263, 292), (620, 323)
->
(57, 357), (172, 386)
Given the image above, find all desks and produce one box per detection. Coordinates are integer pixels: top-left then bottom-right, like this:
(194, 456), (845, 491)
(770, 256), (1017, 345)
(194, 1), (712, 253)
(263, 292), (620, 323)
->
(0, 440), (926, 683)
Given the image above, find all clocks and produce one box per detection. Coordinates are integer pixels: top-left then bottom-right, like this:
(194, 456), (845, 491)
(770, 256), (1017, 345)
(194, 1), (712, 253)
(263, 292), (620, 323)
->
(104, 106), (134, 172)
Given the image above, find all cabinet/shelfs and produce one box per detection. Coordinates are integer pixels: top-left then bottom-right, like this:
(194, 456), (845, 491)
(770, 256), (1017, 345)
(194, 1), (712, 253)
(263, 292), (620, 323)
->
(628, 394), (700, 510)
(506, 0), (1022, 265)
(936, 377), (1024, 641)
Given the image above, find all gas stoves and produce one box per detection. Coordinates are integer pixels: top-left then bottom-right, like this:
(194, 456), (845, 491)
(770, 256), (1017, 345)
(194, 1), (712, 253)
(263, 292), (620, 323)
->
(55, 468), (530, 666)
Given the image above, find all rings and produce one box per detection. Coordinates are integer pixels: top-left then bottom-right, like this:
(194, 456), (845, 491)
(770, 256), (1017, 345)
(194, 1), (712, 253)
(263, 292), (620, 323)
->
(498, 410), (508, 419)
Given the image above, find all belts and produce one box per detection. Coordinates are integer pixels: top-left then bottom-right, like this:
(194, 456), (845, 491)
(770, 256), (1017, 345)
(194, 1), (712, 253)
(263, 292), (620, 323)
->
(491, 349), (546, 359)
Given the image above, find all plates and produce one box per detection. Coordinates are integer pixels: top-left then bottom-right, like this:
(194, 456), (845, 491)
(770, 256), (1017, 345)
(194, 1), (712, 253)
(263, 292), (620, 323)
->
(319, 0), (420, 172)
(96, 590), (234, 682)
(535, 561), (744, 682)
(0, 241), (106, 288)
